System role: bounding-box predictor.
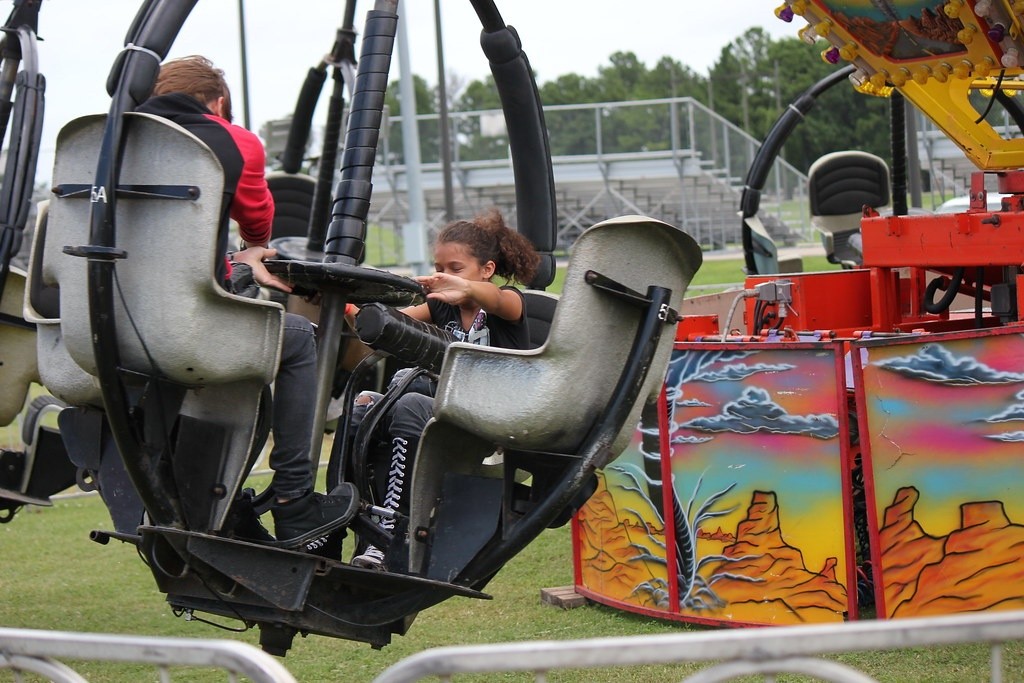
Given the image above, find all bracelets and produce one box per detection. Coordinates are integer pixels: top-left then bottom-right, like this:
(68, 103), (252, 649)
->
(344, 303), (353, 317)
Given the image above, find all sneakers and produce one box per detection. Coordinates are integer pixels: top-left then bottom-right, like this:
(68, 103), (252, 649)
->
(269, 482), (359, 549)
(221, 492), (275, 546)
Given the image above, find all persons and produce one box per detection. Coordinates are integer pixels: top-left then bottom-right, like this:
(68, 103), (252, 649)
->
(303, 210), (539, 570)
(148, 56), (360, 550)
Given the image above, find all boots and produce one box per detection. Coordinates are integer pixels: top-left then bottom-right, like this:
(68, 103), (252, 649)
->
(351, 435), (419, 568)
